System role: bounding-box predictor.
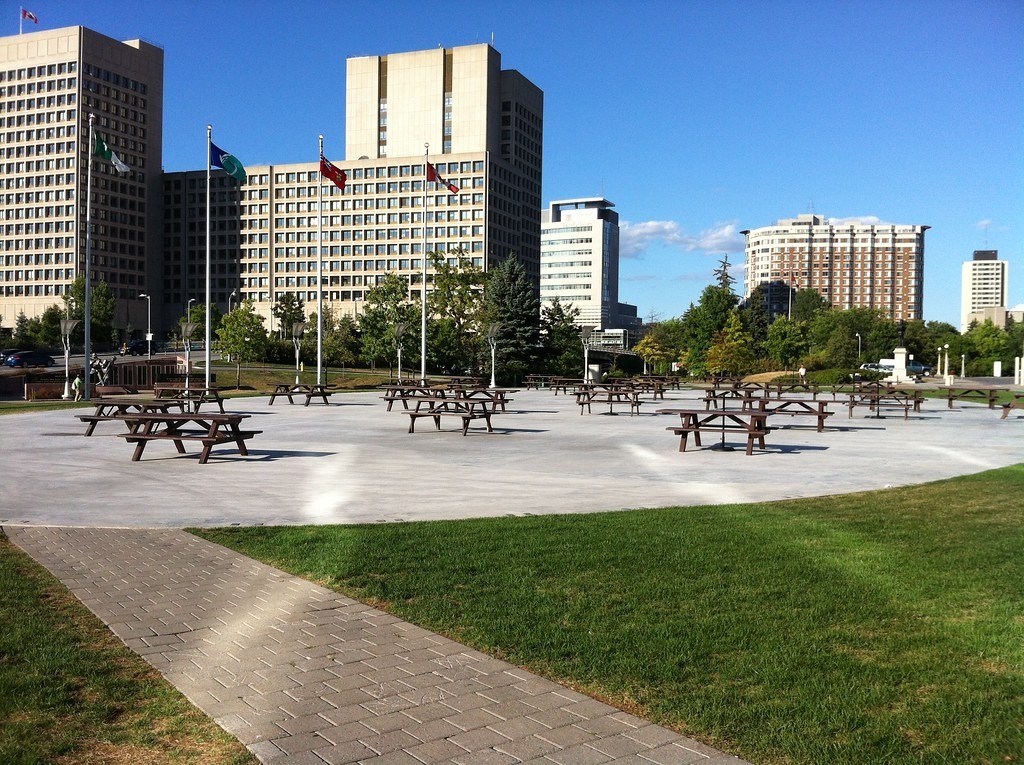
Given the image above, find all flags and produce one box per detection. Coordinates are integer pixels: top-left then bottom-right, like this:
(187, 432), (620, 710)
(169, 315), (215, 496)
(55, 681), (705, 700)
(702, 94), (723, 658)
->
(21, 7), (39, 23)
(210, 142), (246, 181)
(93, 129), (130, 173)
(427, 163), (460, 194)
(319, 152), (347, 190)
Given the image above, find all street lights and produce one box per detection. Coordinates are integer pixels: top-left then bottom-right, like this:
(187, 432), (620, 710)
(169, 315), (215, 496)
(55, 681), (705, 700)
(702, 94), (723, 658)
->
(933, 347), (942, 377)
(139, 294), (150, 360)
(66, 297), (74, 349)
(59, 319), (81, 401)
(227, 289), (237, 362)
(181, 322), (199, 395)
(396, 323), (411, 396)
(292, 323), (308, 392)
(943, 344), (949, 380)
(487, 323), (505, 388)
(856, 332), (861, 360)
(581, 325), (598, 385)
(188, 299), (195, 352)
(960, 355), (966, 377)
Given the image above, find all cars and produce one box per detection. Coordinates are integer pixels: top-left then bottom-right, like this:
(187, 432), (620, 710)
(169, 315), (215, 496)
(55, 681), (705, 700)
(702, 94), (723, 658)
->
(0, 348), (55, 368)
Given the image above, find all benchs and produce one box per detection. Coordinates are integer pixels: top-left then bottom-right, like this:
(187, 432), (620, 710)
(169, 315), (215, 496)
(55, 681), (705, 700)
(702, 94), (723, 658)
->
(522, 381), (1024, 438)
(380, 387), (514, 418)
(260, 392), (336, 396)
(74, 396), (264, 442)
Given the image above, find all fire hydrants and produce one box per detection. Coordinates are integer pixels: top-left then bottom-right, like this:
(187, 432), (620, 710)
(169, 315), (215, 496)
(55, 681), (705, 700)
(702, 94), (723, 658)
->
(300, 361), (304, 372)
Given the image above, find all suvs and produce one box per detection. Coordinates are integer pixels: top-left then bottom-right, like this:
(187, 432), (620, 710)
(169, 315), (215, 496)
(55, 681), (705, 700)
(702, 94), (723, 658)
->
(118, 340), (157, 356)
(859, 359), (934, 377)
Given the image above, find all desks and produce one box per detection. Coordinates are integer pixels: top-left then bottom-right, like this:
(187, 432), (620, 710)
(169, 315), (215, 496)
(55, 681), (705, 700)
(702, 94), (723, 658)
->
(744, 397), (828, 432)
(84, 402), (213, 433)
(763, 383), (823, 400)
(554, 378), (596, 396)
(573, 391), (642, 416)
(573, 383), (635, 403)
(656, 408), (779, 454)
(703, 388), (762, 411)
(153, 386), (226, 414)
(445, 377), (487, 393)
(120, 414), (251, 464)
(267, 383), (336, 406)
(375, 386), (449, 411)
(385, 376), (430, 397)
(408, 399), (494, 436)
(455, 390), (520, 412)
(848, 392), (910, 419)
(526, 376), (557, 390)
(937, 386), (1009, 408)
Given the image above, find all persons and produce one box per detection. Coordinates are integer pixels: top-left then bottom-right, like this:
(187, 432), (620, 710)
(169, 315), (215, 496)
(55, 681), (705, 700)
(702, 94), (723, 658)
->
(123, 343), (126, 352)
(90, 353), (116, 386)
(73, 374), (84, 402)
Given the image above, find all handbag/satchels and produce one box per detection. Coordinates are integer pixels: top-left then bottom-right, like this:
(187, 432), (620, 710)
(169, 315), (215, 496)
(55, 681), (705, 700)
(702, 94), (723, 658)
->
(72, 382), (76, 390)
